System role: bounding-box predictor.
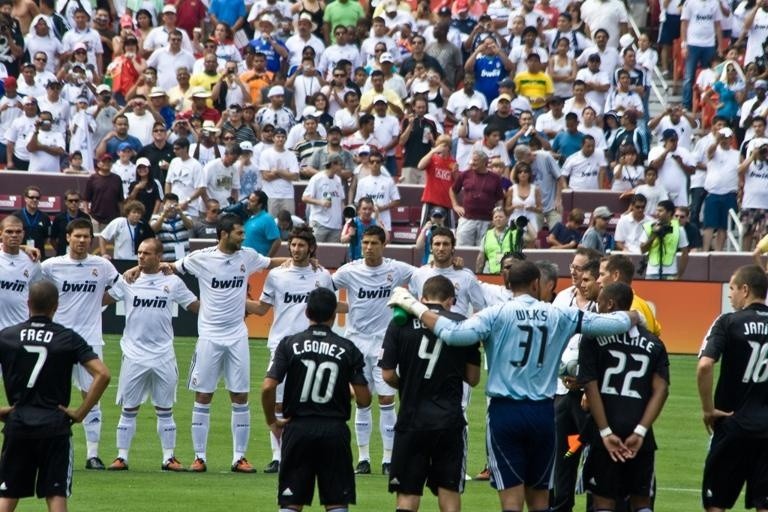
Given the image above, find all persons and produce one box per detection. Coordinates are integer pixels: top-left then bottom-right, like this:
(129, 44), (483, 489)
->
(0, 277), (111, 511)
(691, 264), (767, 512)
(39, 216), (173, 469)
(257, 285), (373, 511)
(121, 212), (324, 474)
(0, 212), (42, 383)
(244, 226), (348, 474)
(329, 226), (464, 475)
(100, 235), (199, 472)
(0, 241), (42, 262)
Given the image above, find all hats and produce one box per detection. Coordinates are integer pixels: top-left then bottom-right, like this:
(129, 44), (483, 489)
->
(3, 6), (679, 219)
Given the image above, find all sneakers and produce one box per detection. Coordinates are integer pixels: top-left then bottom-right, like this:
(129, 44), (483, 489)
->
(83, 455), (131, 471)
(262, 460), (282, 472)
(380, 462), (393, 475)
(161, 454), (208, 471)
(229, 457), (258, 476)
(472, 465), (494, 481)
(353, 459), (373, 475)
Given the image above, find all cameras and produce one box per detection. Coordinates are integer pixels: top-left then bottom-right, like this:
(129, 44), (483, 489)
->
(71, 73), (80, 79)
(167, 203), (175, 209)
(229, 109), (236, 116)
(135, 101), (143, 107)
(413, 115), (420, 120)
(656, 223), (672, 236)
(103, 95), (110, 102)
(144, 75), (152, 81)
(39, 120), (50, 126)
(177, 120), (185, 127)
(228, 70), (234, 74)
(301, 64), (309, 73)
